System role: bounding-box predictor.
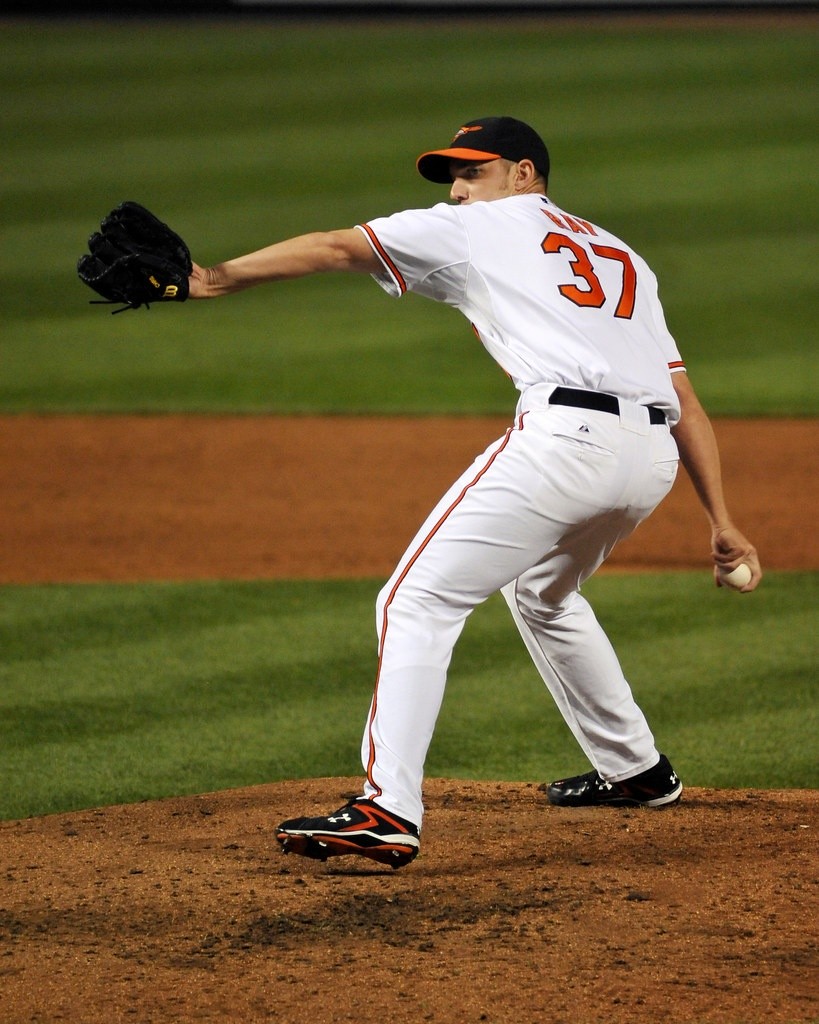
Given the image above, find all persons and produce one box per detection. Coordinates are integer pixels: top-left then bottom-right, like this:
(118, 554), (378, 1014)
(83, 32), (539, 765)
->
(124, 117), (763, 866)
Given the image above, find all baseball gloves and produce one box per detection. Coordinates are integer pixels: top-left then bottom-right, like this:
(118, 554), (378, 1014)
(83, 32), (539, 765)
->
(76, 200), (194, 315)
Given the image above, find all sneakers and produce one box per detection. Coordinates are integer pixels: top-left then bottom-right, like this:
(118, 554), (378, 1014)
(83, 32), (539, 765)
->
(275, 797), (420, 869)
(546, 753), (682, 806)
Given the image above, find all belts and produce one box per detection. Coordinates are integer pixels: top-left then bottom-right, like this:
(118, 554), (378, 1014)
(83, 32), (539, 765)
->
(549, 386), (666, 425)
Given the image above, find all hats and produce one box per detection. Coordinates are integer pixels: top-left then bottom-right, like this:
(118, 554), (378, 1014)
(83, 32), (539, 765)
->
(415, 117), (552, 182)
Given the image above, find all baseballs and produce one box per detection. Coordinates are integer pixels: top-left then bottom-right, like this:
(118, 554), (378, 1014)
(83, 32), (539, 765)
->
(720, 563), (753, 589)
